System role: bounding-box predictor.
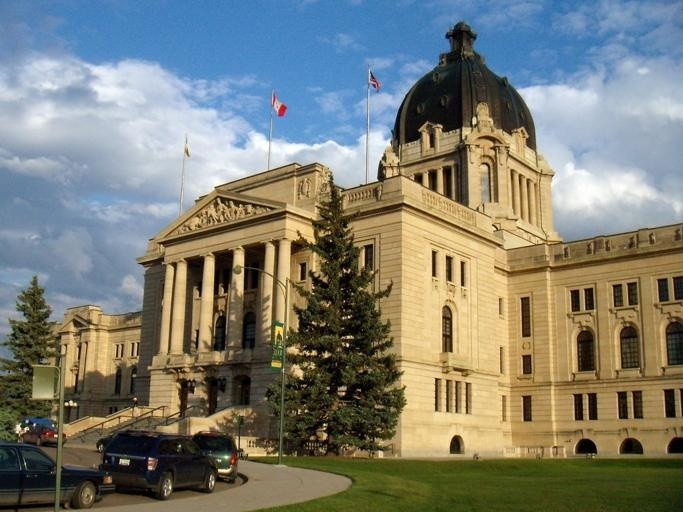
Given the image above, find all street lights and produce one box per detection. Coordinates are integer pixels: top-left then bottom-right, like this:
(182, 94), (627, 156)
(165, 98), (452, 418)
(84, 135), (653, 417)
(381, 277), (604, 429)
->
(233, 264), (288, 465)
(64, 400), (78, 422)
(133, 397), (137, 406)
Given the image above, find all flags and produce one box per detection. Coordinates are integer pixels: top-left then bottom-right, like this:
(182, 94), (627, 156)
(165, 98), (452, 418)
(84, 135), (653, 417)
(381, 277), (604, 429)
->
(368, 69), (380, 93)
(182, 134), (189, 156)
(271, 91), (287, 117)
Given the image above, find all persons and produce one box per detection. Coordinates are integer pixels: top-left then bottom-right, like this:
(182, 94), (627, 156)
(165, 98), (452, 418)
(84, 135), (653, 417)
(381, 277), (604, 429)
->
(174, 198), (272, 234)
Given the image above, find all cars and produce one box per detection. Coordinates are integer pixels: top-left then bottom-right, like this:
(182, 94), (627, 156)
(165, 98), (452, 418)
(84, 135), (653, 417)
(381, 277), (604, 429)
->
(0, 442), (116, 509)
(96, 429), (237, 499)
(21, 425), (67, 446)
